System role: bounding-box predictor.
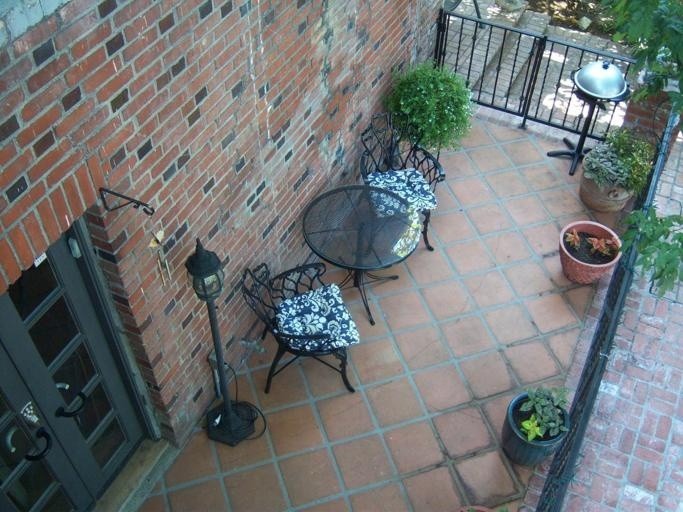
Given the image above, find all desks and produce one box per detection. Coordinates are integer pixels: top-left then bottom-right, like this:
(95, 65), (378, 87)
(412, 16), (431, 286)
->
(299, 185), (421, 327)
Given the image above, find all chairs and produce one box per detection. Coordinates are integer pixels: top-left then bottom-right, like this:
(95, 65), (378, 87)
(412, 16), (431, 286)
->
(354, 111), (446, 264)
(240, 261), (359, 396)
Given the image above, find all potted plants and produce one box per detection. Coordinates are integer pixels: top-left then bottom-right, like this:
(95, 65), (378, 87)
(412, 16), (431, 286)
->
(559, 128), (656, 285)
(500, 382), (571, 468)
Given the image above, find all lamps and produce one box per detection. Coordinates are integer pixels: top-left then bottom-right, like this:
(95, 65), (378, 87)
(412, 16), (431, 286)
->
(183, 235), (256, 446)
